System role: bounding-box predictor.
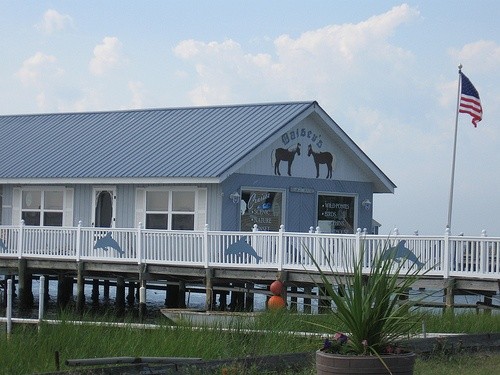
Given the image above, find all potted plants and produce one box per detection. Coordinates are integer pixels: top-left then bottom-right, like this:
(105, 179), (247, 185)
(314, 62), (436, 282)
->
(290, 231), (457, 375)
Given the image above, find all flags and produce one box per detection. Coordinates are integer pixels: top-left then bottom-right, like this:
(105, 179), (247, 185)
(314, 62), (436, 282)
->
(458, 69), (483, 127)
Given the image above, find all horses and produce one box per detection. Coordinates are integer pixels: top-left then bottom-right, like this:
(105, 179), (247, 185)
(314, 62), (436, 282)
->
(271, 142), (301, 176)
(307, 142), (334, 179)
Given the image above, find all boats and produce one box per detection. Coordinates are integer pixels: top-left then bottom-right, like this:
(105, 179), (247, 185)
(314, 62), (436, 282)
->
(161, 305), (339, 330)
